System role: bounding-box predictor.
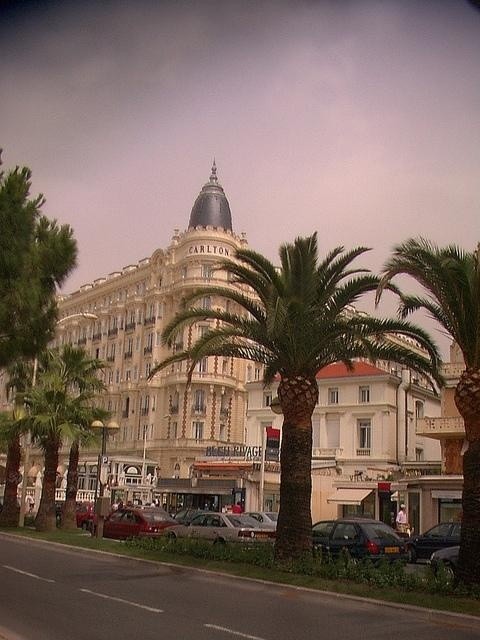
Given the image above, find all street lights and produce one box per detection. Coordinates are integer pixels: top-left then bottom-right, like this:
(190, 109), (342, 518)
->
(270, 398), (285, 414)
(90, 420), (121, 538)
(17, 312), (98, 529)
(141, 413), (173, 499)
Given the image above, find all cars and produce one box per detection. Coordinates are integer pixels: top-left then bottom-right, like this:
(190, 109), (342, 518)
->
(430, 545), (460, 588)
(310, 519), (403, 566)
(163, 512), (277, 550)
(244, 510), (279, 527)
(54, 499), (223, 542)
(403, 520), (463, 563)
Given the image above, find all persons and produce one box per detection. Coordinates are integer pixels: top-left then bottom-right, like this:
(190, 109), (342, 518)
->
(395, 503), (408, 533)
(27, 493), (35, 513)
(233, 502), (242, 514)
(150, 495), (159, 508)
(118, 501), (123, 510)
